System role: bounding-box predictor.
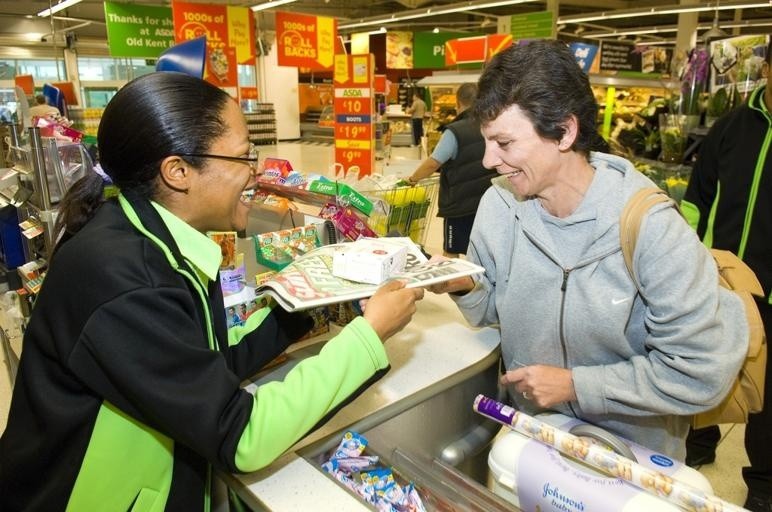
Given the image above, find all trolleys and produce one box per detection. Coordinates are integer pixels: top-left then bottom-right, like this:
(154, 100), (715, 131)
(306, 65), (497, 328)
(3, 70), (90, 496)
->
(375, 137), (393, 167)
(353, 172), (441, 247)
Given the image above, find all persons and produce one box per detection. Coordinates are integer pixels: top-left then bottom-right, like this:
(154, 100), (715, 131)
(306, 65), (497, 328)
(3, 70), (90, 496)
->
(29, 95), (61, 127)
(405, 92), (427, 148)
(425, 37), (751, 470)
(245, 300), (258, 318)
(227, 306), (242, 328)
(404, 82), (498, 259)
(674, 35), (772, 511)
(260, 297), (268, 308)
(239, 303), (247, 321)
(0, 68), (427, 512)
(219, 233), (233, 267)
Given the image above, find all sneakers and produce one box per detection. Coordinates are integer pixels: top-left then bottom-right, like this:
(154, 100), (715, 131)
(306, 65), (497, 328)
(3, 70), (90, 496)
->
(683, 444), (718, 468)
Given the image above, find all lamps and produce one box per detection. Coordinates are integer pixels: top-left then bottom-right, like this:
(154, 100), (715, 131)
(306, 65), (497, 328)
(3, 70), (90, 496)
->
(700, 1), (729, 38)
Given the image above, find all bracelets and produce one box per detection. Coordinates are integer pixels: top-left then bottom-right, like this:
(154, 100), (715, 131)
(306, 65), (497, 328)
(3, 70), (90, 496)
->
(409, 177), (417, 185)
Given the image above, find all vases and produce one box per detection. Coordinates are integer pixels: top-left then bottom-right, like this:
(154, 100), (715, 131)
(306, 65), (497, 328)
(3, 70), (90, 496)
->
(657, 112), (701, 164)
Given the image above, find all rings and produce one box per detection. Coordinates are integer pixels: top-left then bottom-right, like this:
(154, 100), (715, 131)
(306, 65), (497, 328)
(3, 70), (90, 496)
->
(522, 391), (530, 400)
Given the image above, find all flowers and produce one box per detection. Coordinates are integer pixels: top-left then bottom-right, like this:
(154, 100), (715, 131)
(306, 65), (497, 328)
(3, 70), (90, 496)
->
(658, 46), (711, 157)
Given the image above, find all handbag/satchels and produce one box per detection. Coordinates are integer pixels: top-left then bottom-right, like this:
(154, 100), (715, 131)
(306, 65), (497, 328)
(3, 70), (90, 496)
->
(616, 184), (769, 433)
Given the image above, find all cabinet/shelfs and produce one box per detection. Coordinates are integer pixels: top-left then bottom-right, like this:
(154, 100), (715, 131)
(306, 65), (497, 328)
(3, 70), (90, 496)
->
(8, 126), (95, 262)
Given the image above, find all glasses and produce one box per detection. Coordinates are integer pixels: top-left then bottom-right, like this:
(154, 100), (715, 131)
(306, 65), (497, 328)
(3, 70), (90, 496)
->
(156, 142), (260, 172)
